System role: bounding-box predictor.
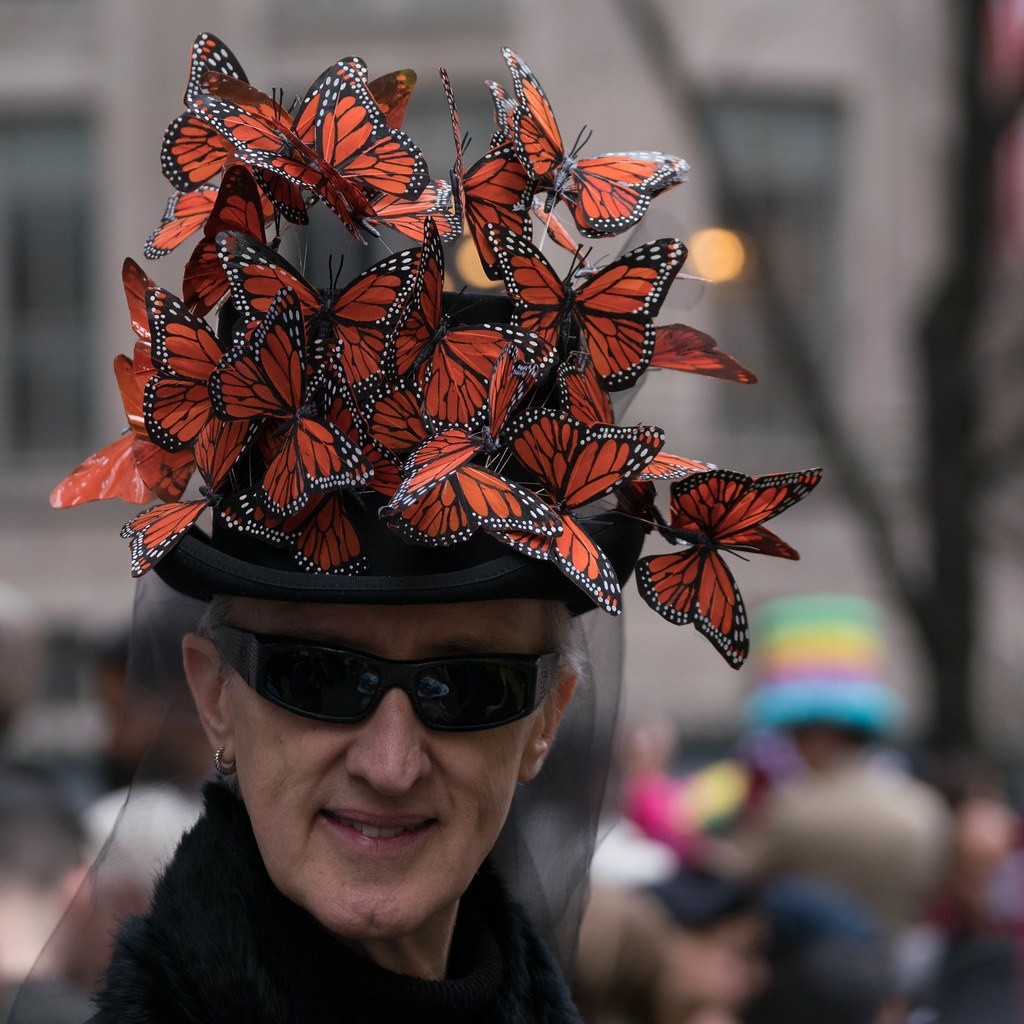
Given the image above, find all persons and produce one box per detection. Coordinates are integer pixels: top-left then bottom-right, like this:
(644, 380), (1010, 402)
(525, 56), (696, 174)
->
(0, 595), (1024, 1024)
(85, 288), (650, 1024)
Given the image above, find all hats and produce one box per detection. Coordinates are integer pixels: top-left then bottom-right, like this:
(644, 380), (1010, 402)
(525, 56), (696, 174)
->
(149, 289), (646, 619)
(744, 593), (902, 740)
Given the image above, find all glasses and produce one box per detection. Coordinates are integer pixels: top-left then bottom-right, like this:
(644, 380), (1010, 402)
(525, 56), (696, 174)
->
(214, 625), (565, 733)
